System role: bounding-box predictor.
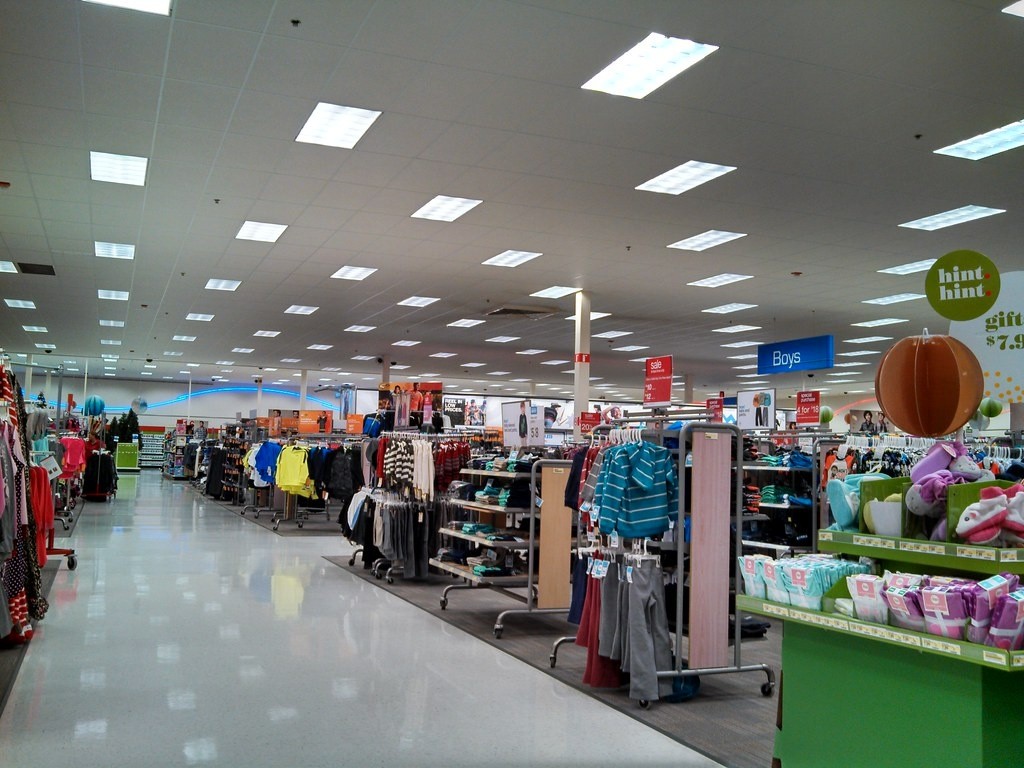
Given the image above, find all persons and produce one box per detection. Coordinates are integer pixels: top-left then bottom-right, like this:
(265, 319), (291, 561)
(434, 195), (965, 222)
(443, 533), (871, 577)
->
(186, 421), (195, 434)
(406, 382), (423, 411)
(293, 410), (299, 418)
(85, 431), (104, 460)
(753, 392), (771, 427)
(195, 421), (207, 440)
(469, 399), (488, 425)
(519, 402), (528, 446)
(859, 411), (874, 434)
(390, 385), (404, 405)
(317, 411), (327, 433)
(273, 410), (282, 430)
(603, 406), (622, 425)
(874, 411), (887, 435)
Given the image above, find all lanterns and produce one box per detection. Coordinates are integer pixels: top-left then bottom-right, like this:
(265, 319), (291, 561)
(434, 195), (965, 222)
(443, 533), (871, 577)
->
(981, 397), (1003, 417)
(877, 332), (984, 438)
(83, 396), (106, 416)
(819, 405), (833, 424)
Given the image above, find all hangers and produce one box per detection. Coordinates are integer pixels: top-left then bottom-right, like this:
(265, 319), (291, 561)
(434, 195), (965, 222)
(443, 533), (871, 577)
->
(838, 435), (1024, 468)
(258, 429), (501, 509)
(577, 427), (665, 569)
(0, 349), (113, 468)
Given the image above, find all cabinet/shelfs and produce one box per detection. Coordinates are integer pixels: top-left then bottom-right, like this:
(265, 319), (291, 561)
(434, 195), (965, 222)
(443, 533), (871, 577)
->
(642, 428), (813, 555)
(429, 470), (540, 585)
(164, 434), (193, 477)
(736, 476), (1024, 671)
(138, 436), (164, 467)
